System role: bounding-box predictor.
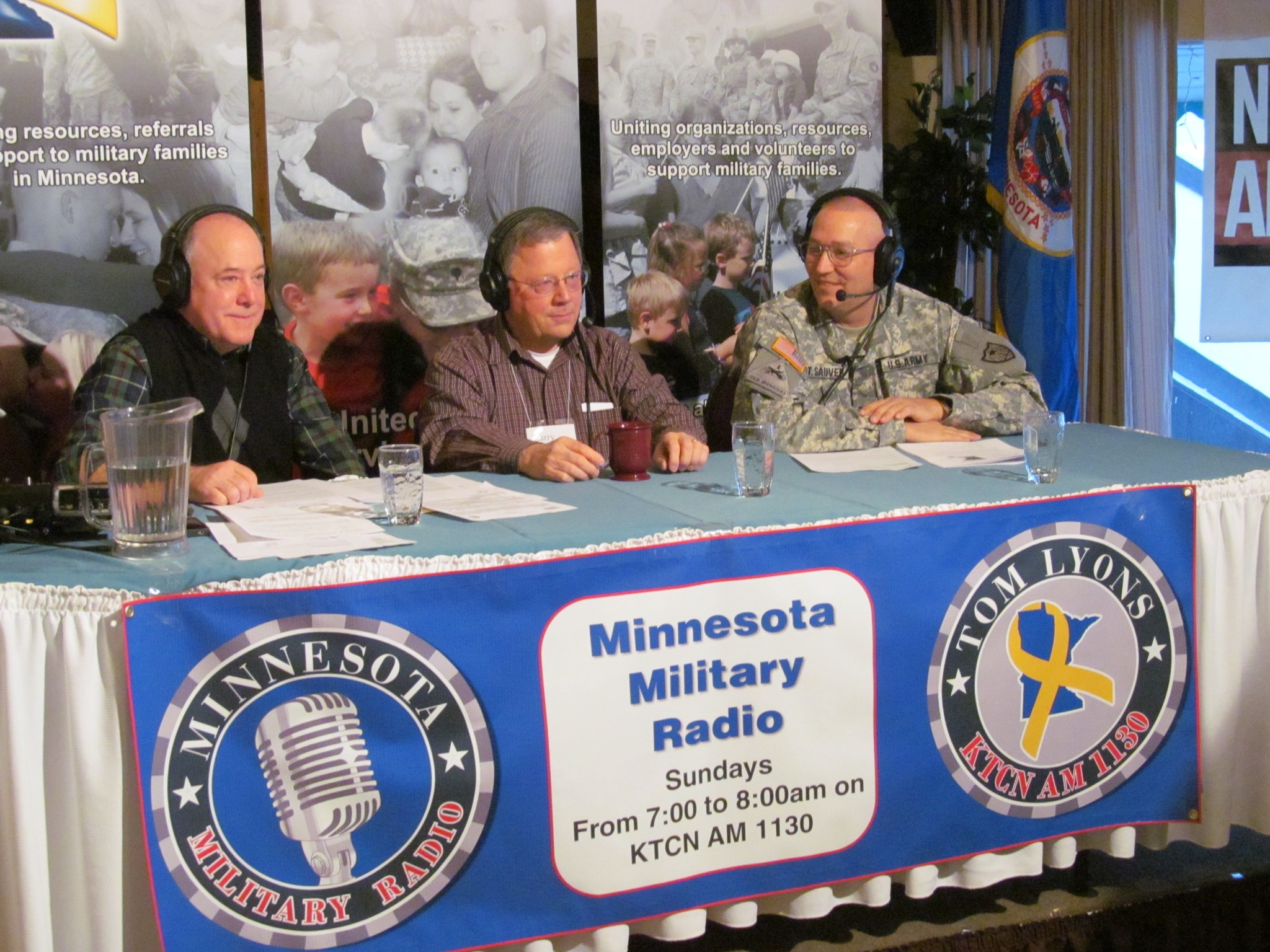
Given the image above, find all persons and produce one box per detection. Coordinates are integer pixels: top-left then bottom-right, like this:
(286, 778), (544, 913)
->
(52, 204), (366, 515)
(724, 188), (1050, 454)
(414, 208), (710, 487)
(1, 1), (884, 474)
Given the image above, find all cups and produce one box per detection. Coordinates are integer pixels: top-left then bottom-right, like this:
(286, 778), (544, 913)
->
(1021, 409), (1065, 482)
(377, 443), (424, 526)
(606, 418), (655, 480)
(732, 420), (776, 496)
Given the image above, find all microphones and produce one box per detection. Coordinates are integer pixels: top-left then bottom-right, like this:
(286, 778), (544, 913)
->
(262, 284), (283, 330)
(835, 257), (902, 302)
(582, 281), (601, 328)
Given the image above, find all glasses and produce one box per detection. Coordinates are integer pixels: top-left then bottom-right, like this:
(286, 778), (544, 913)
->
(507, 269), (589, 296)
(797, 241), (875, 266)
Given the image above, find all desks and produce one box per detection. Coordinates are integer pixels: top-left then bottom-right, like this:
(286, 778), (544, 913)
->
(1, 421), (1269, 952)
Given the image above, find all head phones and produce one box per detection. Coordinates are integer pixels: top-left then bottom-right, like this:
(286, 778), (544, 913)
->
(477, 205), (593, 309)
(153, 201), (273, 309)
(798, 185), (905, 288)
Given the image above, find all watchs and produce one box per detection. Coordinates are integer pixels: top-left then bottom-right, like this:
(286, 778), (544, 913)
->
(934, 396), (952, 421)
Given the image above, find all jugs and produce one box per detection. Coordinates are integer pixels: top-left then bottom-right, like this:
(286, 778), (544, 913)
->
(81, 394), (205, 562)
